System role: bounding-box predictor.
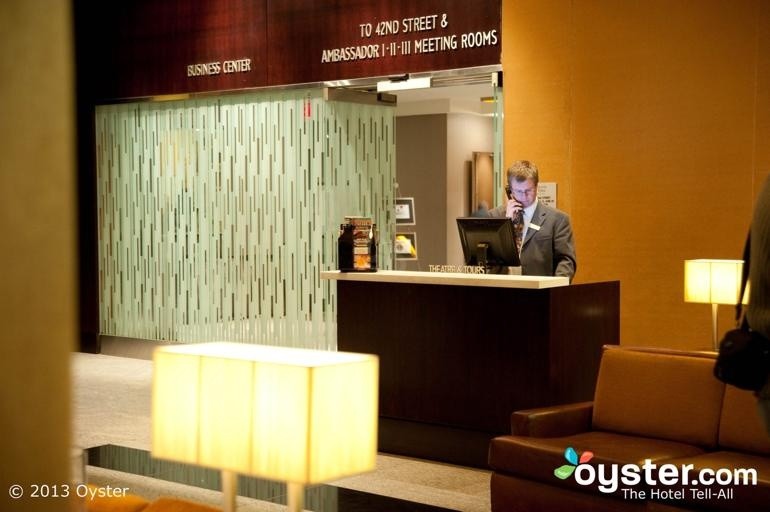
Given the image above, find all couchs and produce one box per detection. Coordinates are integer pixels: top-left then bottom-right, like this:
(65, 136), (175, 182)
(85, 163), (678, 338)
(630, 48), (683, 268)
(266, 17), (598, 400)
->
(487, 342), (770, 512)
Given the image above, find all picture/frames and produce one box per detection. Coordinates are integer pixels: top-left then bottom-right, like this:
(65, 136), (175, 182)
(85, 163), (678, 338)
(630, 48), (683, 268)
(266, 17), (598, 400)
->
(396, 231), (418, 261)
(392, 197), (417, 227)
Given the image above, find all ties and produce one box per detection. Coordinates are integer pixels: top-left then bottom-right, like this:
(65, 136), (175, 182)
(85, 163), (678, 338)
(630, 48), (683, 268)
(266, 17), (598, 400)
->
(512, 209), (524, 254)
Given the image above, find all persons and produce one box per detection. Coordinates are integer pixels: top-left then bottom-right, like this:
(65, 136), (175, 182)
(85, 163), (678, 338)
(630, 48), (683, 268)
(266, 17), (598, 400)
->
(746, 172), (769, 434)
(486, 160), (576, 285)
(471, 200), (490, 219)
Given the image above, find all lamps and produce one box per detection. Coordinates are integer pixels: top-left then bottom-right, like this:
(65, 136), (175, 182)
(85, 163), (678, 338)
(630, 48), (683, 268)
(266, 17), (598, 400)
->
(148, 340), (384, 512)
(682, 256), (752, 350)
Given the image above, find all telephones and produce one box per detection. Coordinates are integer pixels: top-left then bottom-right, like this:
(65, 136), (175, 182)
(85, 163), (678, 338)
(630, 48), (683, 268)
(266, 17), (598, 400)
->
(505, 185), (523, 212)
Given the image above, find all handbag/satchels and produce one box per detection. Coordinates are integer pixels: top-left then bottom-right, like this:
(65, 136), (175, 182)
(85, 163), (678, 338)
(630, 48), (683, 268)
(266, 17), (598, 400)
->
(713, 328), (769, 391)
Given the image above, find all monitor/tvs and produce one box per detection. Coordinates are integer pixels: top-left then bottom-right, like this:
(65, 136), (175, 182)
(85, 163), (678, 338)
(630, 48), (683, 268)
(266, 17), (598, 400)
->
(455, 216), (522, 268)
